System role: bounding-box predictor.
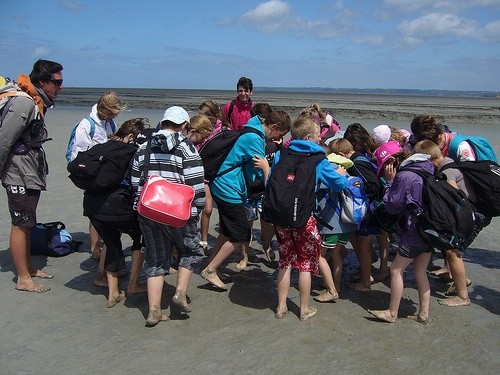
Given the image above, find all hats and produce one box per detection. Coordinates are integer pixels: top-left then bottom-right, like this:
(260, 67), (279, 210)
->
(161, 106), (190, 127)
(372, 125), (391, 144)
(372, 141), (403, 178)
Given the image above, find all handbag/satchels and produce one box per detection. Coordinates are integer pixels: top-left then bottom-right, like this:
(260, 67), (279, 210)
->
(135, 173), (197, 227)
(30, 221), (83, 257)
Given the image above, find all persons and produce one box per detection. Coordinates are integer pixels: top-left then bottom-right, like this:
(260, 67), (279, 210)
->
(199, 110), (292, 291)
(369, 114), (500, 323)
(251, 103), (380, 319)
(67, 91), (227, 326)
(223, 77), (257, 130)
(0, 59), (64, 293)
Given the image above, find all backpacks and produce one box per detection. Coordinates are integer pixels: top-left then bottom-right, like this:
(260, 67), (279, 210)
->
(67, 140), (137, 192)
(441, 156), (500, 216)
(397, 166), (476, 252)
(356, 157), (398, 232)
(340, 175), (368, 224)
(199, 125), (265, 184)
(258, 146), (327, 229)
(448, 130), (497, 162)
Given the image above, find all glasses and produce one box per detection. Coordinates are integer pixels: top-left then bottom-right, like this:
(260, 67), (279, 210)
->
(49, 78), (63, 86)
